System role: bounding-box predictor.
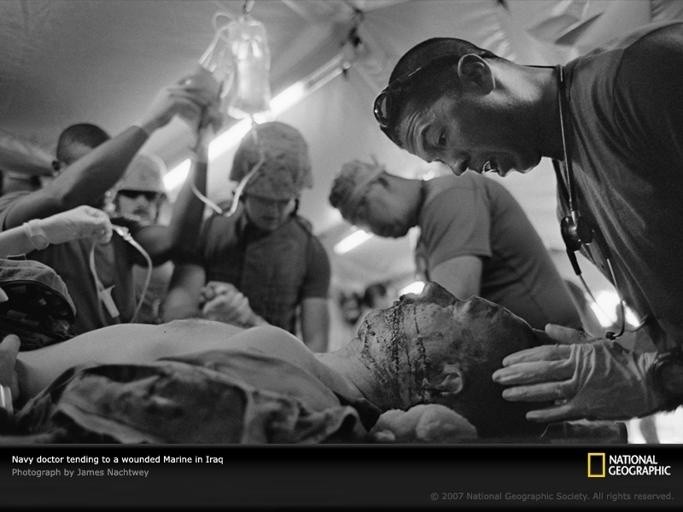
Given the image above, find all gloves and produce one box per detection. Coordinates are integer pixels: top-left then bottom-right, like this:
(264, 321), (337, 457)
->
(132, 72), (218, 153)
(493, 321), (671, 426)
(24, 205), (113, 251)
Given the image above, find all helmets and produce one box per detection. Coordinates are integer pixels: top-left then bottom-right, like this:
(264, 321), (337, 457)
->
(228, 120), (314, 202)
(106, 149), (170, 205)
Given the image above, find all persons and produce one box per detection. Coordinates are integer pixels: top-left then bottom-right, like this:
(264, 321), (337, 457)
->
(0, 19), (683, 447)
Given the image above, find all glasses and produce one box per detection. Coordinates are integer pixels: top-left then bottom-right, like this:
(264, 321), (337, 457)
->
(373, 54), (450, 141)
